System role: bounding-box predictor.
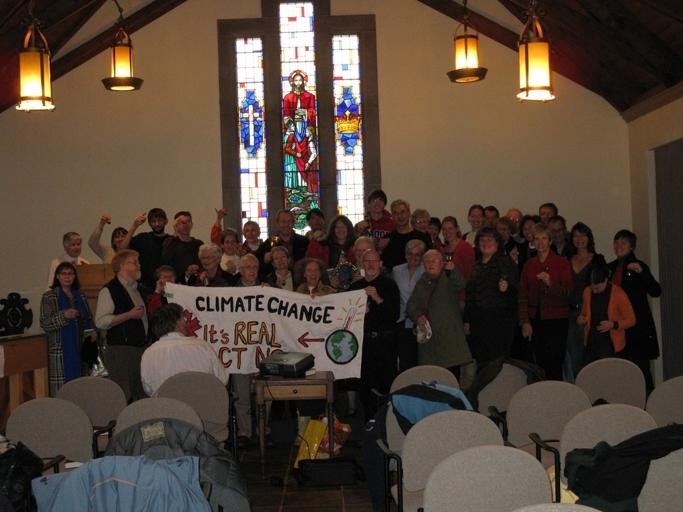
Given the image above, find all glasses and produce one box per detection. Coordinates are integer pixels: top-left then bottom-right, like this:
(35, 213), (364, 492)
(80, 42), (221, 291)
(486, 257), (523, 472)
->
(424, 259), (441, 266)
(127, 260), (138, 267)
(59, 272), (74, 278)
(508, 216), (519, 222)
(241, 265), (258, 270)
(415, 220), (427, 224)
(179, 220), (191, 225)
(178, 317), (187, 323)
(160, 275), (176, 281)
(199, 255), (216, 260)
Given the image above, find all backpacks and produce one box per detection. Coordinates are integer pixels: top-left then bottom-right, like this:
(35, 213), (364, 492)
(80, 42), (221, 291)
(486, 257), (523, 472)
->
(391, 380), (481, 438)
(562, 422), (682, 503)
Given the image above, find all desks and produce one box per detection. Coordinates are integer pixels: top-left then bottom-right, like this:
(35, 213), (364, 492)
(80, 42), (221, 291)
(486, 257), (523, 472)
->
(253, 369), (338, 480)
(1, 333), (51, 414)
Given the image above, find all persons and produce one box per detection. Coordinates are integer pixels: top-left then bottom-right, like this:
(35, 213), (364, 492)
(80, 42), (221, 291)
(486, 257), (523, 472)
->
(45, 230), (90, 290)
(91, 250), (152, 406)
(148, 188), (663, 454)
(285, 107), (320, 198)
(40, 260), (100, 396)
(280, 70), (317, 193)
(139, 301), (228, 397)
(87, 213), (127, 262)
(119, 206), (176, 291)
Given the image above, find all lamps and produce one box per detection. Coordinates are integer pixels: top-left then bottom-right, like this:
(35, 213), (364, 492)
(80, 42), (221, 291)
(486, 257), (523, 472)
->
(12, 2), (58, 115)
(98, 1), (143, 94)
(445, 0), (559, 107)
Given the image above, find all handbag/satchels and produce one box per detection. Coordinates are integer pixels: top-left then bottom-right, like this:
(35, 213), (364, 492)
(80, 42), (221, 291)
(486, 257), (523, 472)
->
(292, 411), (353, 470)
(87, 345), (110, 378)
(288, 456), (367, 489)
(416, 320), (432, 344)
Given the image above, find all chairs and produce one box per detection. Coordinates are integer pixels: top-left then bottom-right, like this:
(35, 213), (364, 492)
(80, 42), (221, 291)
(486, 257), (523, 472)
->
(0, 369), (250, 512)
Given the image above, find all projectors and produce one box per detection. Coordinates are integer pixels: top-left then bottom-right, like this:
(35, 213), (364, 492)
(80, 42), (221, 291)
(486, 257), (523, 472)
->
(259, 352), (314, 376)
